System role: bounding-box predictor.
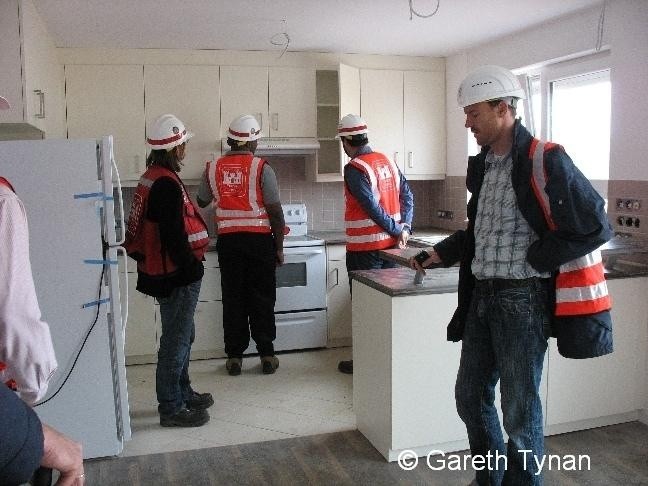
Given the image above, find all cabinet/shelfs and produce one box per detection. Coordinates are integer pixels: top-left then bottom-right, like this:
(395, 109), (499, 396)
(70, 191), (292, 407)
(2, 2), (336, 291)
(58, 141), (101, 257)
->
(144, 64), (222, 179)
(65, 64), (145, 181)
(219, 64), (317, 152)
(359, 68), (448, 181)
(120, 248), (224, 366)
(0, 1), (50, 135)
(327, 243), (355, 350)
(303, 62), (359, 184)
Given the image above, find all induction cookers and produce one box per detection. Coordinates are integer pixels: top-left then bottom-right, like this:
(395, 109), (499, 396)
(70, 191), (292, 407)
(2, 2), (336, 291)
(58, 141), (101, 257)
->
(279, 202), (325, 247)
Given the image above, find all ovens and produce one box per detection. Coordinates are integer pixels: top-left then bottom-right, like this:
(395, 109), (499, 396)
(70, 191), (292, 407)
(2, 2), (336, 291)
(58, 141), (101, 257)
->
(243, 245), (328, 356)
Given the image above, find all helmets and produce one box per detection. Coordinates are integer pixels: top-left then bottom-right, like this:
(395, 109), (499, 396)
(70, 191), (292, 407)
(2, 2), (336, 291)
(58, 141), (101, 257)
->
(336, 114), (367, 139)
(457, 66), (526, 107)
(145, 114), (192, 150)
(228, 114), (262, 142)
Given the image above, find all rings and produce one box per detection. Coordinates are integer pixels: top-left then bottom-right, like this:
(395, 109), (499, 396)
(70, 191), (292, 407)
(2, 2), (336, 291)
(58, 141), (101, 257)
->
(76, 473), (85, 480)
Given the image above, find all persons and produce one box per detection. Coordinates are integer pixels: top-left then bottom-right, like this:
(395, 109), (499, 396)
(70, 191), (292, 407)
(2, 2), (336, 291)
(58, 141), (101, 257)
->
(1, 382), (87, 485)
(334, 112), (416, 374)
(120, 112), (214, 428)
(1, 175), (60, 404)
(408, 62), (615, 485)
(195, 115), (286, 376)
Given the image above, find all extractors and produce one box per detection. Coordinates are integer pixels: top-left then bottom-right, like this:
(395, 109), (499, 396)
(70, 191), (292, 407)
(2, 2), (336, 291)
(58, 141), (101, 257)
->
(220, 137), (322, 156)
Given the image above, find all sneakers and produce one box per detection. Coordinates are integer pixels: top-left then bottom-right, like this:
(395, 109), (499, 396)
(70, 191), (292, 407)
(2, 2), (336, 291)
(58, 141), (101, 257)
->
(226, 358), (243, 375)
(186, 392), (214, 409)
(261, 355), (279, 374)
(338, 360), (353, 374)
(160, 407), (209, 428)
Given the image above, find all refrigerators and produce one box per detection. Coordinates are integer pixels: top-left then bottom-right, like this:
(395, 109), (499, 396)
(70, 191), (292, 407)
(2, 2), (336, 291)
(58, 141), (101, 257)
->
(1, 135), (134, 463)
